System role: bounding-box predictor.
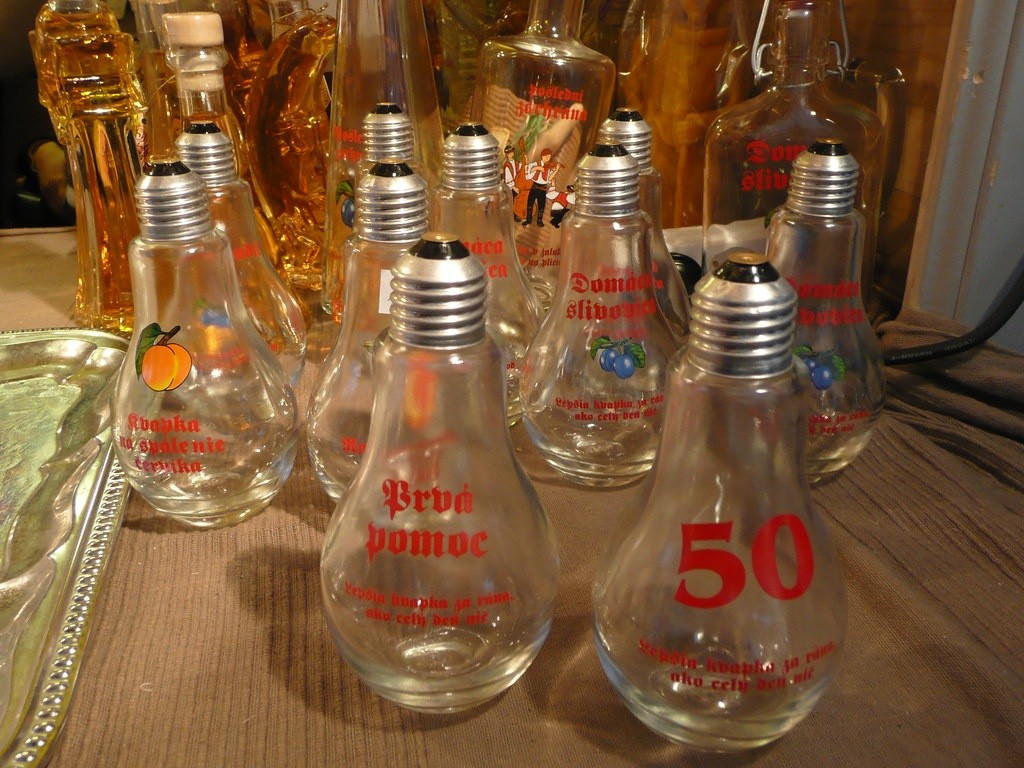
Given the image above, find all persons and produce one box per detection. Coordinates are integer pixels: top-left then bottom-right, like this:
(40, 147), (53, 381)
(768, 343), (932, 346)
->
(0, 0), (67, 207)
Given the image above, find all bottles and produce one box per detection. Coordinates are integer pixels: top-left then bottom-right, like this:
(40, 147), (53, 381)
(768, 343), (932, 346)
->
(160, 10), (312, 337)
(320, 0), (444, 314)
(702, 0), (884, 275)
(29, 0), (151, 339)
(469, 0), (616, 309)
